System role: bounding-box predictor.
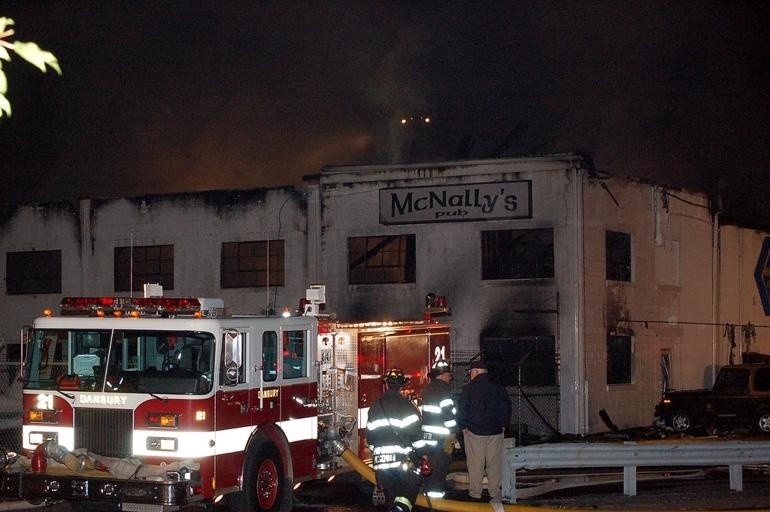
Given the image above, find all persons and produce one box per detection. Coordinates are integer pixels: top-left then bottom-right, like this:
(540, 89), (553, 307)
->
(420, 359), (462, 498)
(460, 360), (514, 501)
(368, 367), (429, 512)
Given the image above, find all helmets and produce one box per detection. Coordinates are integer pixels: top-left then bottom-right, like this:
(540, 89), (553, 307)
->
(381, 367), (409, 385)
(427, 360), (454, 378)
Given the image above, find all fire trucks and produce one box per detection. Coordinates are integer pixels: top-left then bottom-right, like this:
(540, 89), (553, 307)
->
(1, 284), (455, 511)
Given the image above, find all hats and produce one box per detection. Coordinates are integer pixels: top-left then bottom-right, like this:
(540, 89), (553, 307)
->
(467, 360), (489, 371)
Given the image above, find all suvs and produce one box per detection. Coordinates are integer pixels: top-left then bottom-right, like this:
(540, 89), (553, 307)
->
(654, 363), (770, 434)
